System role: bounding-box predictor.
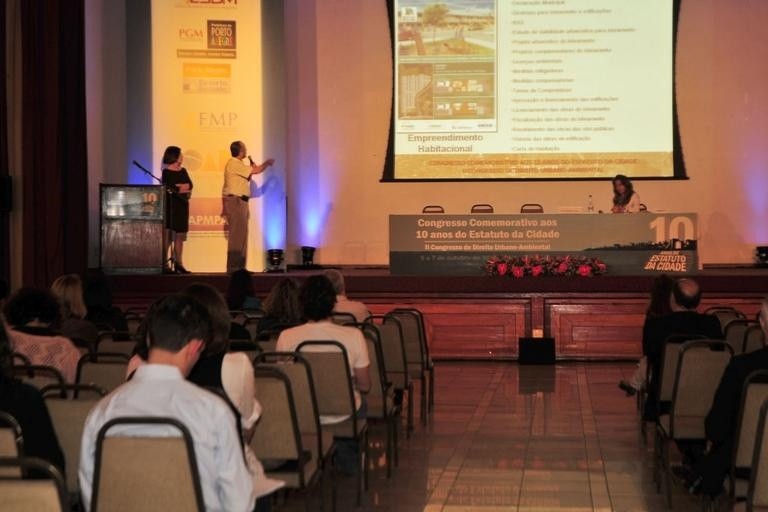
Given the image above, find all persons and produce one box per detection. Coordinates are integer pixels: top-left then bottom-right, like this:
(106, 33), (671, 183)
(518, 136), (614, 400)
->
(227, 269), (305, 342)
(616, 274), (675, 396)
(640, 278), (726, 489)
(50, 273), (129, 341)
(667, 296), (768, 502)
(276, 274), (372, 457)
(79, 282), (262, 512)
(5, 286), (80, 400)
(161, 144), (193, 272)
(324, 269), (370, 324)
(222, 138), (275, 272)
(609, 174), (640, 213)
(0, 320), (65, 480)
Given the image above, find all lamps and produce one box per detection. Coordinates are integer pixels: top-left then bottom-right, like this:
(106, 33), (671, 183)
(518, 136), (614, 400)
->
(302, 245), (315, 264)
(266, 245), (284, 269)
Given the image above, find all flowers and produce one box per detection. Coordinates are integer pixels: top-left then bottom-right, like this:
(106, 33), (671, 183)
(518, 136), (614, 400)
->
(486, 253), (606, 276)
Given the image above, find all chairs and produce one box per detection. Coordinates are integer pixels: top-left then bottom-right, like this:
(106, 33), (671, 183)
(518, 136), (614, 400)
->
(472, 204), (493, 213)
(423, 204), (443, 213)
(635, 307), (767, 511)
(521, 203), (544, 213)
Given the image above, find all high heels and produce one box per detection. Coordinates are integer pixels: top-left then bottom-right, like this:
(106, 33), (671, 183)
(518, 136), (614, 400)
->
(174, 261), (191, 274)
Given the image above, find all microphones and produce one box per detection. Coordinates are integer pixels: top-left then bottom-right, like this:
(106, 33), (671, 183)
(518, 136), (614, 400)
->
(247, 155), (256, 167)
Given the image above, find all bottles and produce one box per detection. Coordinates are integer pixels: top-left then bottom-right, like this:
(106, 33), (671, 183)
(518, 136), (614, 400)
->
(588, 194), (593, 212)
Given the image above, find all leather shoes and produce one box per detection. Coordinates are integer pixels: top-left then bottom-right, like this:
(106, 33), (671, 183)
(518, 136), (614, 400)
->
(618, 382), (636, 397)
